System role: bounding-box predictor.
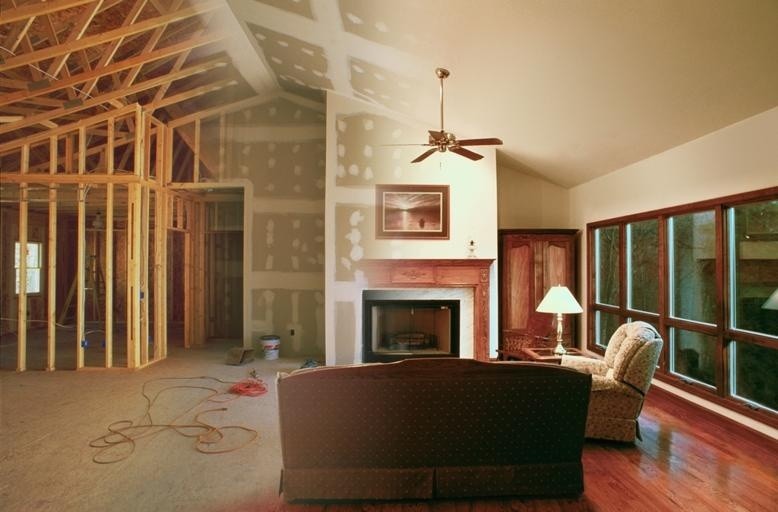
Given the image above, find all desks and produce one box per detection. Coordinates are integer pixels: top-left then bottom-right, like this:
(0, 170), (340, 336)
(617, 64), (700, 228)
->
(523, 346), (596, 365)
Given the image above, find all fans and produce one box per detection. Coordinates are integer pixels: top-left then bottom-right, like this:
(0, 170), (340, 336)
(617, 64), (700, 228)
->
(380, 67), (504, 164)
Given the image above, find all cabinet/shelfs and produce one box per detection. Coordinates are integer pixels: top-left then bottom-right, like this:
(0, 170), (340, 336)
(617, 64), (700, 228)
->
(499, 228), (579, 361)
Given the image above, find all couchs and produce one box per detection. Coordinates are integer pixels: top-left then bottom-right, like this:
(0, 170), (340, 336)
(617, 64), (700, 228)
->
(274, 357), (593, 502)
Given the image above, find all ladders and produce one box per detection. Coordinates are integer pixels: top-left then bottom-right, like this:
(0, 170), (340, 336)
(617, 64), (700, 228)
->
(58, 255), (106, 330)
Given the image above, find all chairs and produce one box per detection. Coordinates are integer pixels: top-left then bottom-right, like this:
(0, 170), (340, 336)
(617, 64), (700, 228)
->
(495, 311), (573, 361)
(560, 320), (664, 443)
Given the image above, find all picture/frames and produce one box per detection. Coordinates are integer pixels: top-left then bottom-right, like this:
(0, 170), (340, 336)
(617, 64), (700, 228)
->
(373, 183), (452, 240)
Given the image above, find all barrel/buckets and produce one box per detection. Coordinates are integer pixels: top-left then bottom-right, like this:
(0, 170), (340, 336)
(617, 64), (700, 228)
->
(260, 335), (280, 360)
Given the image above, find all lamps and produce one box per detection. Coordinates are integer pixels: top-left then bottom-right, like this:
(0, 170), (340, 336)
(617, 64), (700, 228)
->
(535, 282), (584, 355)
(92, 211), (103, 229)
